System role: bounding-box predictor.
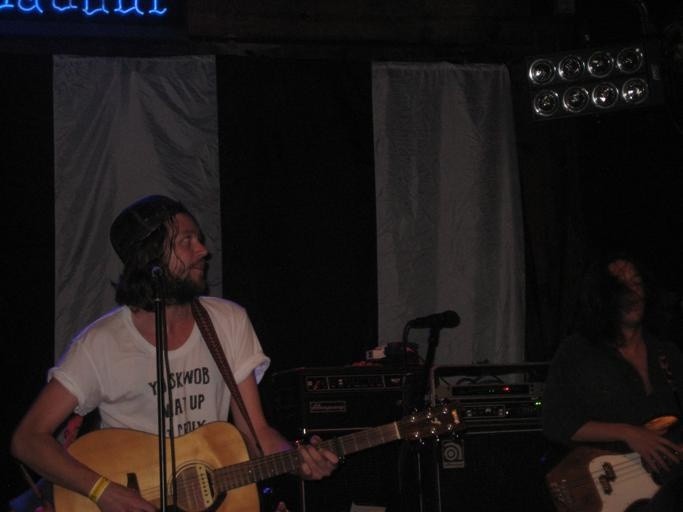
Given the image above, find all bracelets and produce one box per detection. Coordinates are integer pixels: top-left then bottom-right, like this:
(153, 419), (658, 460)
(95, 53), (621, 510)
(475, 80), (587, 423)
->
(87, 474), (112, 503)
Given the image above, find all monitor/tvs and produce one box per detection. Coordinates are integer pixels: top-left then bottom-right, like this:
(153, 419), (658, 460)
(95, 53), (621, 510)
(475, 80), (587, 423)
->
(0, 1), (229, 57)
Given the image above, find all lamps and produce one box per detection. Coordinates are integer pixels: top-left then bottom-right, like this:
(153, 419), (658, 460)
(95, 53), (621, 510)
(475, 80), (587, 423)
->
(509, 41), (665, 130)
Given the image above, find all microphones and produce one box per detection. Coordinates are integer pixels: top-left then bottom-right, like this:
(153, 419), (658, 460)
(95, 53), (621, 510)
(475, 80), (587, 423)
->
(409, 310), (460, 329)
(152, 266), (163, 300)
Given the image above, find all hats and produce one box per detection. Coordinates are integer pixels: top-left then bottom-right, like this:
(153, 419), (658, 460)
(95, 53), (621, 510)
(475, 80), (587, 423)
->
(110, 194), (186, 265)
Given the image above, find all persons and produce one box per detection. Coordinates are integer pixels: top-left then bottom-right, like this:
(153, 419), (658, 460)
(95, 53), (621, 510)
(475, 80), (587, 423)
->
(10, 191), (340, 511)
(537, 227), (683, 511)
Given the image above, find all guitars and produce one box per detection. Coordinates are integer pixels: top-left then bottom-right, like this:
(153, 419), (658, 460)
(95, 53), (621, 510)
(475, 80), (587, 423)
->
(544, 416), (683, 512)
(53, 402), (461, 512)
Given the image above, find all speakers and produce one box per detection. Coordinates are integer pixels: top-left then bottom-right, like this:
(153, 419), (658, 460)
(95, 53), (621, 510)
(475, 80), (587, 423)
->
(282, 427), (423, 512)
(433, 428), (556, 512)
